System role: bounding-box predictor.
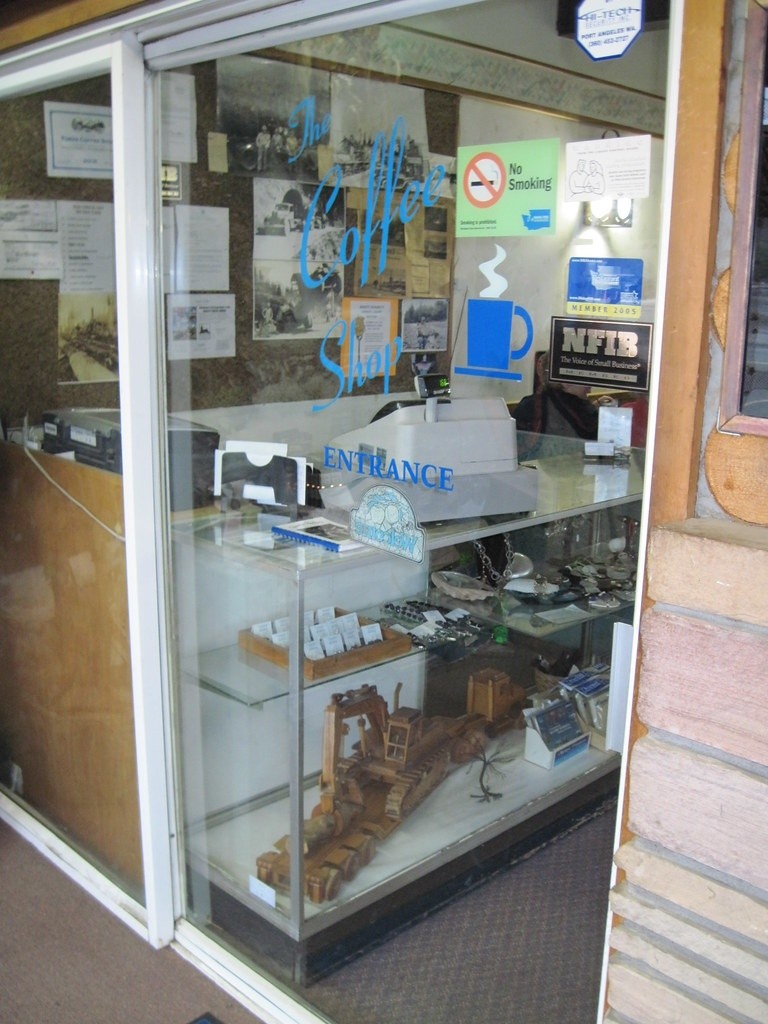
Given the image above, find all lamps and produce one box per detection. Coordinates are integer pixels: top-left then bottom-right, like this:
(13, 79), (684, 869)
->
(583, 128), (634, 229)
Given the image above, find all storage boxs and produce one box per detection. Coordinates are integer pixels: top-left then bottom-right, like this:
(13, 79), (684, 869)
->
(524, 713), (593, 770)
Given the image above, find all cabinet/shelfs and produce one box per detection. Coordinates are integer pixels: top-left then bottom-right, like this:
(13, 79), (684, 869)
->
(170, 446), (646, 990)
